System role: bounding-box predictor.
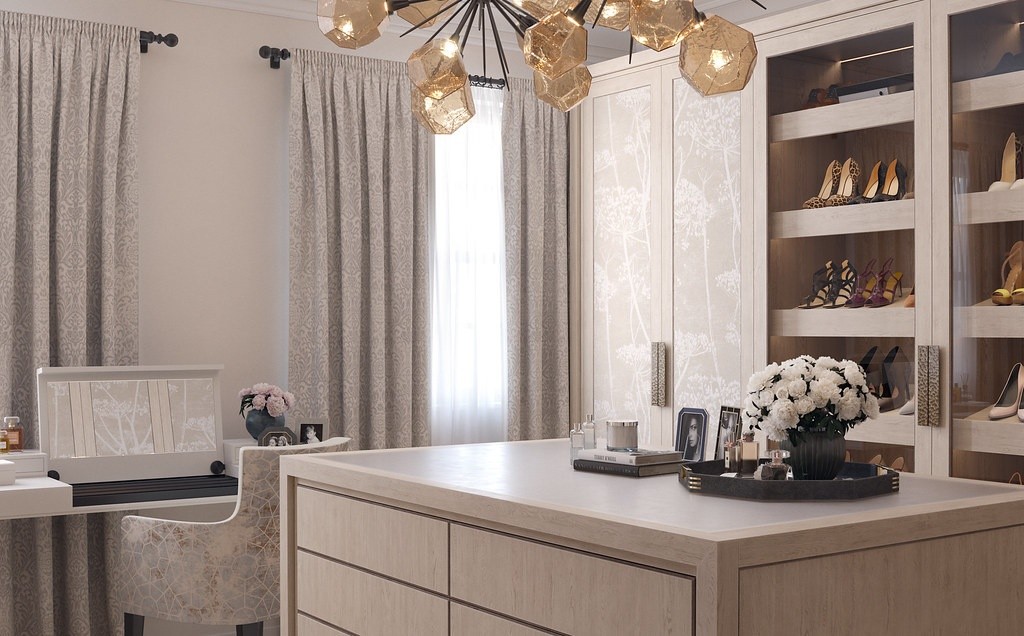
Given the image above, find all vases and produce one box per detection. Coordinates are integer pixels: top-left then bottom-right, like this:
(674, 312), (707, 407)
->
(788, 433), (847, 480)
(241, 408), (290, 441)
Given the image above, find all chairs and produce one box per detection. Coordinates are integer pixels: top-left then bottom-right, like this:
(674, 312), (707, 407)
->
(116, 438), (352, 636)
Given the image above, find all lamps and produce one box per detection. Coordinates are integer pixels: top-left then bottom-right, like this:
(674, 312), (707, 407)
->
(316, 1), (763, 135)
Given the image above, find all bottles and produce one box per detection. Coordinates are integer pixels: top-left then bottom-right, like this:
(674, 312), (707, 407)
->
(761, 450), (793, 481)
(570, 423), (585, 465)
(1, 417), (24, 451)
(0, 431), (9, 453)
(724, 433), (742, 472)
(738, 431), (759, 474)
(582, 414), (595, 450)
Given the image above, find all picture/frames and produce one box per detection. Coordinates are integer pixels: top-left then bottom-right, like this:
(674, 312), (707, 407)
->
(716, 406), (742, 463)
(257, 426), (297, 447)
(294, 416), (331, 444)
(675, 409), (710, 462)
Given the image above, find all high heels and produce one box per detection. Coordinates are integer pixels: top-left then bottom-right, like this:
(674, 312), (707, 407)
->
(875, 346), (912, 412)
(859, 346), (884, 397)
(991, 240), (1024, 304)
(988, 132), (1024, 192)
(802, 88), (824, 109)
(844, 259), (879, 307)
(819, 84), (840, 105)
(864, 257), (903, 309)
(798, 260), (840, 309)
(826, 157), (862, 205)
(988, 363), (1024, 420)
(871, 158), (908, 202)
(805, 160), (843, 208)
(848, 161), (888, 205)
(823, 259), (858, 309)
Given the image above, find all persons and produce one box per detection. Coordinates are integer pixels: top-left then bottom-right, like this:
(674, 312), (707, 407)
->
(269, 436), (288, 446)
(307, 425), (320, 444)
(682, 414), (701, 461)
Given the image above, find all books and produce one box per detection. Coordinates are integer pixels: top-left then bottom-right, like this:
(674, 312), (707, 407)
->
(574, 448), (685, 477)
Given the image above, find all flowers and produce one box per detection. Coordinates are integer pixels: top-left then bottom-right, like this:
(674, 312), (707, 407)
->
(237, 382), (299, 417)
(729, 342), (886, 440)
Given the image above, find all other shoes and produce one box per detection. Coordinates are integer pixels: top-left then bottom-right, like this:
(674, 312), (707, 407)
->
(869, 454), (887, 467)
(845, 450), (851, 462)
(1008, 472), (1023, 484)
(899, 397), (915, 414)
(904, 282), (915, 308)
(891, 457), (909, 472)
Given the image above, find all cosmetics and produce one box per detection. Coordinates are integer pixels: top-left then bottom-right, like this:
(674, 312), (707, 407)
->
(761, 451), (793, 480)
(724, 432), (759, 478)
(0, 416), (23, 453)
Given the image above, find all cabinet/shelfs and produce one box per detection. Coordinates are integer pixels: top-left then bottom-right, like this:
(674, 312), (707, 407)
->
(731, 0), (1024, 493)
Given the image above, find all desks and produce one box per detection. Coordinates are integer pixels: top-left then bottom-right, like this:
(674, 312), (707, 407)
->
(280, 435), (1024, 636)
